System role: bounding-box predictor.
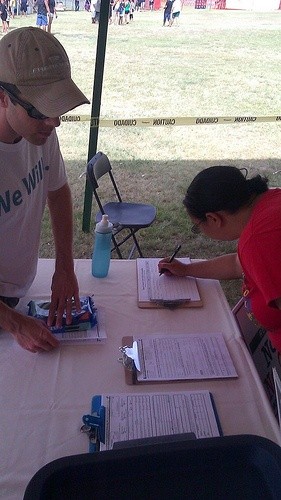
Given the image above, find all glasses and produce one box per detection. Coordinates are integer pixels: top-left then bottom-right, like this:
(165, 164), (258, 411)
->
(0, 83), (49, 120)
(191, 218), (209, 235)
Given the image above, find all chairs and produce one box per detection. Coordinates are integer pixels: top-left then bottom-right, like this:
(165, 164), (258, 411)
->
(84, 148), (159, 260)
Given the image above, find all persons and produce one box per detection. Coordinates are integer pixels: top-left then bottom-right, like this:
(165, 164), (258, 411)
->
(0, 0), (184, 32)
(0, 26), (91, 353)
(158, 165), (281, 355)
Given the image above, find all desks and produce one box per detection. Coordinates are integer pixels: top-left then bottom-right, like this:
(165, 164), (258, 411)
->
(0, 255), (281, 500)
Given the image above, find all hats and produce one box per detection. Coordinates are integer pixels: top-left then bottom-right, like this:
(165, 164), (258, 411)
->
(0, 26), (90, 118)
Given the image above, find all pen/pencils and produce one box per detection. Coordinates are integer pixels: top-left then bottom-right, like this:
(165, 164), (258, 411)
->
(158, 244), (182, 276)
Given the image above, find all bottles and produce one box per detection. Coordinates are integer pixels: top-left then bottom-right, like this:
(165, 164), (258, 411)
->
(92, 215), (114, 277)
